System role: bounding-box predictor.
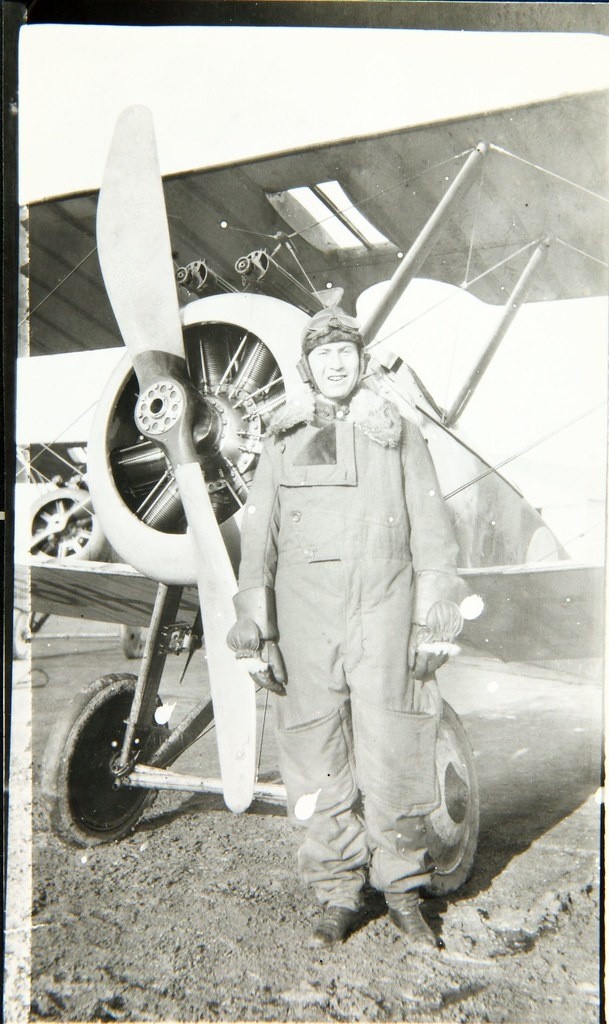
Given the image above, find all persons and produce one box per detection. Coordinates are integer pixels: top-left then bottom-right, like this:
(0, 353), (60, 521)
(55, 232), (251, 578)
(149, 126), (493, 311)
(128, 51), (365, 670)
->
(235, 311), (461, 951)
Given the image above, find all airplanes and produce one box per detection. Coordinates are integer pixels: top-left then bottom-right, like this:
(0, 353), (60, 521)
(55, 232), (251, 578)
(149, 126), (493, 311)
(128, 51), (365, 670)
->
(32, 101), (609, 897)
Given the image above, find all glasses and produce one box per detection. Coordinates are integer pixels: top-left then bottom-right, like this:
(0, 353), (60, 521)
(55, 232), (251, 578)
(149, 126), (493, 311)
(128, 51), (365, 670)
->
(305, 312), (361, 341)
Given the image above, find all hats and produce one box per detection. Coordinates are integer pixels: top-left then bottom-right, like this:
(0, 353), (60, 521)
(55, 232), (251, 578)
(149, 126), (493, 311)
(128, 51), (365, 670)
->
(297, 306), (371, 393)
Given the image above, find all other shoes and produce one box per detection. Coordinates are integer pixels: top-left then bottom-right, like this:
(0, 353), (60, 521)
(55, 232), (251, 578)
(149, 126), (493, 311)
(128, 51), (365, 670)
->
(388, 902), (438, 952)
(310, 906), (368, 947)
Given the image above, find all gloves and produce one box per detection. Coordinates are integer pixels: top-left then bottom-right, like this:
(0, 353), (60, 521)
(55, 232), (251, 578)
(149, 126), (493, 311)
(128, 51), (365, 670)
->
(409, 571), (471, 680)
(226, 584), (285, 692)
